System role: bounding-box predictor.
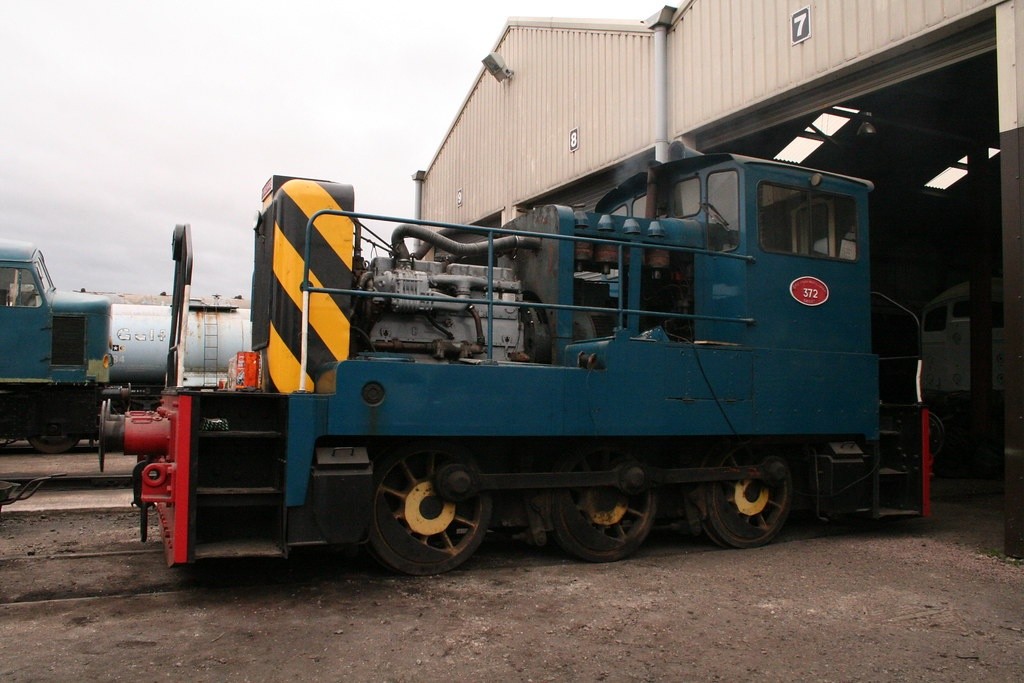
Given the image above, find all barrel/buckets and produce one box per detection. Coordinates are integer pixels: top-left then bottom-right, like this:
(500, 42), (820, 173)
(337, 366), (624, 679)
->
(219, 377), (228, 389)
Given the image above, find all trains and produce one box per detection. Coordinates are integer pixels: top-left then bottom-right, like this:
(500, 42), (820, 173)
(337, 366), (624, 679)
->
(1, 252), (265, 453)
(105, 149), (933, 571)
